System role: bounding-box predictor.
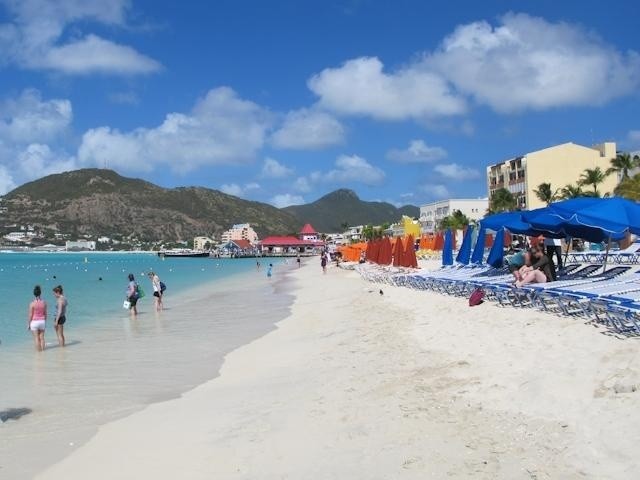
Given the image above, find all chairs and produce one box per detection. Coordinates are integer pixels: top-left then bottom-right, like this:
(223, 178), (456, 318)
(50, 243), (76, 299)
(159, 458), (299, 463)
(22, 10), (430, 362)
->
(352, 243), (640, 338)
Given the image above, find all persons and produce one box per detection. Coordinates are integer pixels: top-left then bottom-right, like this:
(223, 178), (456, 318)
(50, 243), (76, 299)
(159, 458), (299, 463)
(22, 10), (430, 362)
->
(52, 285), (67, 347)
(321, 251), (328, 271)
(125, 274), (139, 317)
(27, 285), (47, 352)
(516, 243), (557, 288)
(267, 264), (273, 278)
(148, 271), (163, 312)
(509, 248), (544, 282)
(545, 236), (564, 270)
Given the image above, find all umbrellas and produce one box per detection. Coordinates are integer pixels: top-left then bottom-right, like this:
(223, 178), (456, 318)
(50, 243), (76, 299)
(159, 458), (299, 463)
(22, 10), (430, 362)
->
(547, 198), (640, 273)
(478, 210), (566, 253)
(520, 206), (627, 269)
(336, 224), (546, 269)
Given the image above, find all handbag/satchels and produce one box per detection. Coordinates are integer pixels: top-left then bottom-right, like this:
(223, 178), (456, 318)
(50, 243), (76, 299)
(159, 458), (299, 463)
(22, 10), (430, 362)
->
(469, 288), (486, 306)
(159, 282), (166, 291)
(137, 286), (145, 298)
(123, 301), (132, 309)
